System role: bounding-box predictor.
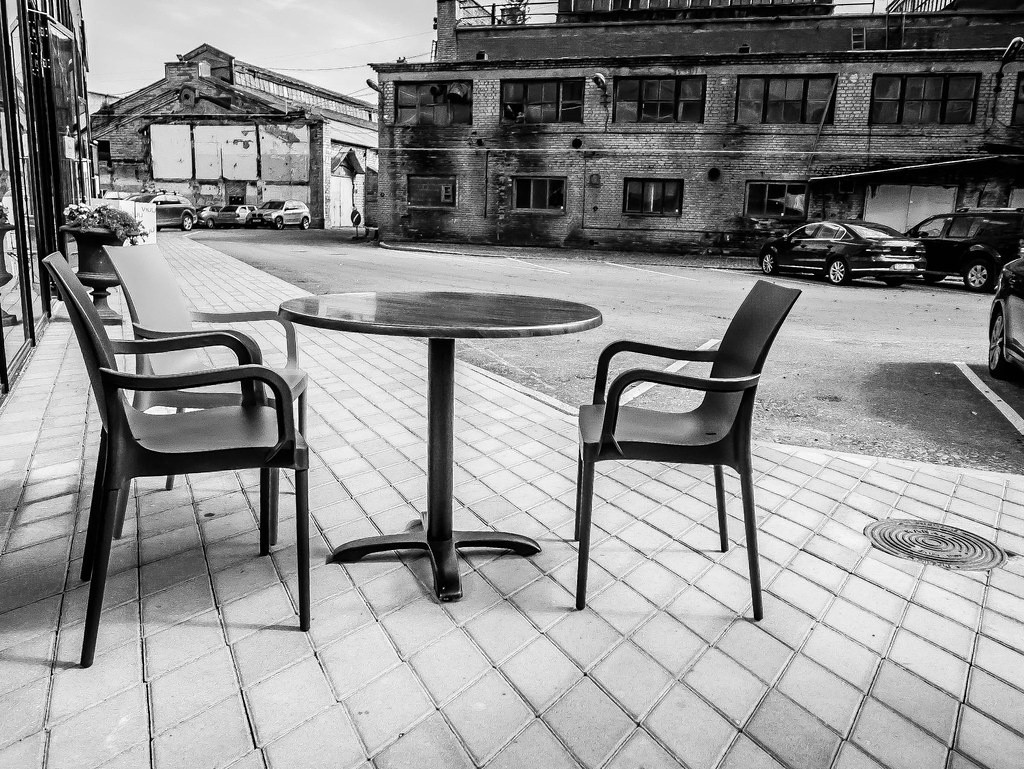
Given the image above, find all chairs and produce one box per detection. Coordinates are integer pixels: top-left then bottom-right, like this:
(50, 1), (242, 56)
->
(41, 250), (310, 669)
(102, 242), (310, 546)
(575, 280), (802, 622)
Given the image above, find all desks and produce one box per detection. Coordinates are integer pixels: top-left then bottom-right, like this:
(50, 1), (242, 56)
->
(278, 290), (603, 603)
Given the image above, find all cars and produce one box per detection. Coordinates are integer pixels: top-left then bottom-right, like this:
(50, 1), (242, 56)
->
(123, 188), (196, 231)
(757, 220), (927, 287)
(989, 258), (1024, 384)
(246, 198), (312, 229)
(217, 204), (257, 229)
(197, 204), (222, 229)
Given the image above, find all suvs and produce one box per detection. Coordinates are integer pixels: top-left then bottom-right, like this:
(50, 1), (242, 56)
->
(902, 206), (1023, 291)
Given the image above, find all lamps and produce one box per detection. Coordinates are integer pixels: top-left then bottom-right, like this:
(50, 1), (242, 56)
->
(592, 72), (611, 104)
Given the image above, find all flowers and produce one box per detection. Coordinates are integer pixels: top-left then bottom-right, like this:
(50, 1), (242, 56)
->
(0, 201), (11, 226)
(63, 201), (149, 246)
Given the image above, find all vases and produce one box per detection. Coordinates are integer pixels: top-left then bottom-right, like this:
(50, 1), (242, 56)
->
(0, 225), (18, 328)
(59, 225), (128, 325)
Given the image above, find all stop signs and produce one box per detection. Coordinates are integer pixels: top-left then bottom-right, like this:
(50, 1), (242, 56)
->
(350, 210), (361, 225)
